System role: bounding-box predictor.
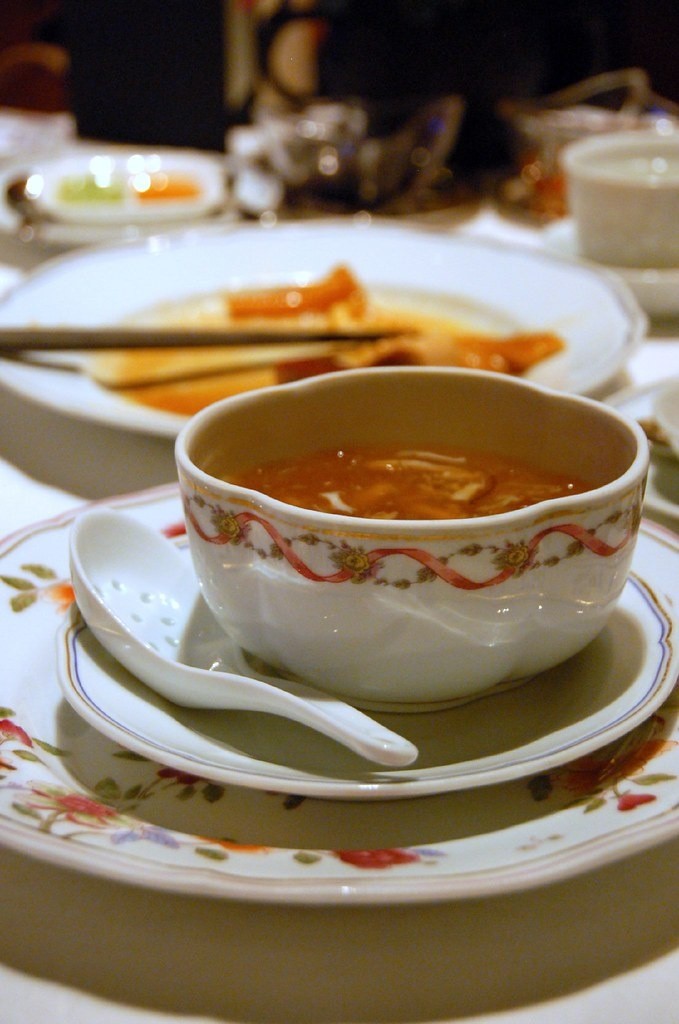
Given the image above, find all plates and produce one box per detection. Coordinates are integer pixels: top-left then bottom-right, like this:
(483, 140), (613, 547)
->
(56, 565), (679, 803)
(0, 159), (282, 246)
(0, 480), (679, 904)
(0, 220), (651, 441)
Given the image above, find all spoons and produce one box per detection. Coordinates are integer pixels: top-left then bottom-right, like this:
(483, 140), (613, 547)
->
(68, 509), (419, 768)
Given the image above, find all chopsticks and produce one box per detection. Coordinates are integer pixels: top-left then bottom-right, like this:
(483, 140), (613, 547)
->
(0, 326), (419, 352)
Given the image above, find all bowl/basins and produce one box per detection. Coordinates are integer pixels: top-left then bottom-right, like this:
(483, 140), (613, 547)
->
(28, 149), (227, 228)
(172, 366), (651, 705)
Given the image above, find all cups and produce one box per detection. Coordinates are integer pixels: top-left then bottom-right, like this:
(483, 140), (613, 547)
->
(556, 130), (679, 269)
